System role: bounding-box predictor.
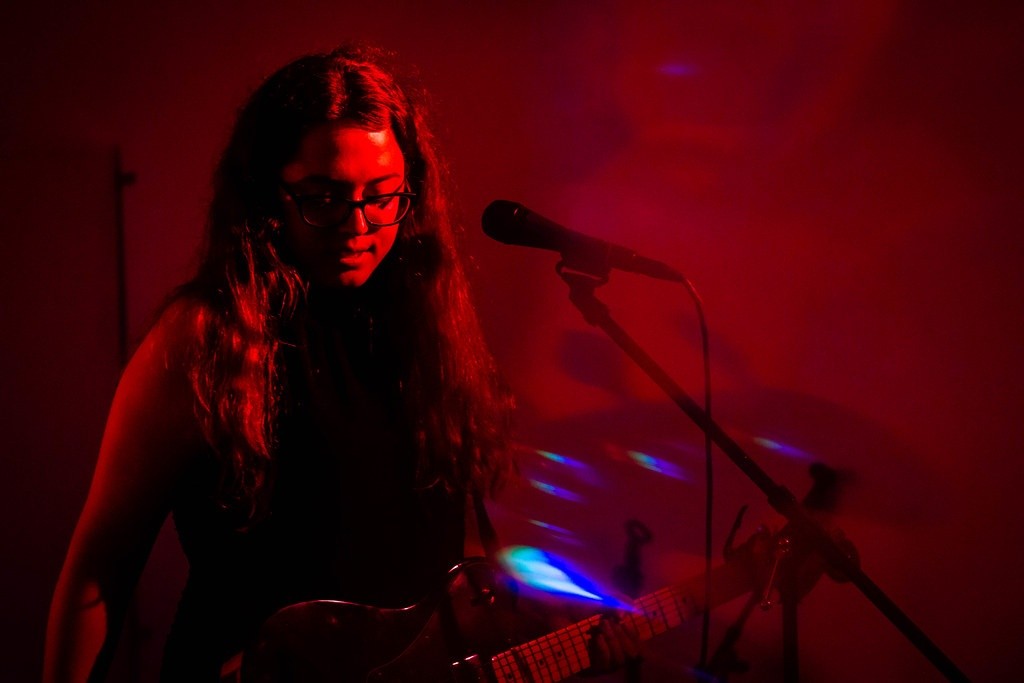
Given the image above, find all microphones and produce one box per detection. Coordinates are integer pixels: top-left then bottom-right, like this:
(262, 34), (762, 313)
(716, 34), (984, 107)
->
(482, 200), (686, 282)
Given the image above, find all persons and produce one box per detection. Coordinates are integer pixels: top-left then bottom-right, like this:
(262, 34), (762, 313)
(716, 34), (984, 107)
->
(43, 45), (636, 683)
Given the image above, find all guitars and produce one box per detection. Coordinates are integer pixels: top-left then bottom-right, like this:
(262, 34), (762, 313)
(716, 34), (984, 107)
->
(152, 521), (859, 683)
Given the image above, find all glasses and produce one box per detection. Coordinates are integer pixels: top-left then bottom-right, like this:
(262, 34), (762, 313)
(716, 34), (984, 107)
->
(279, 180), (417, 230)
